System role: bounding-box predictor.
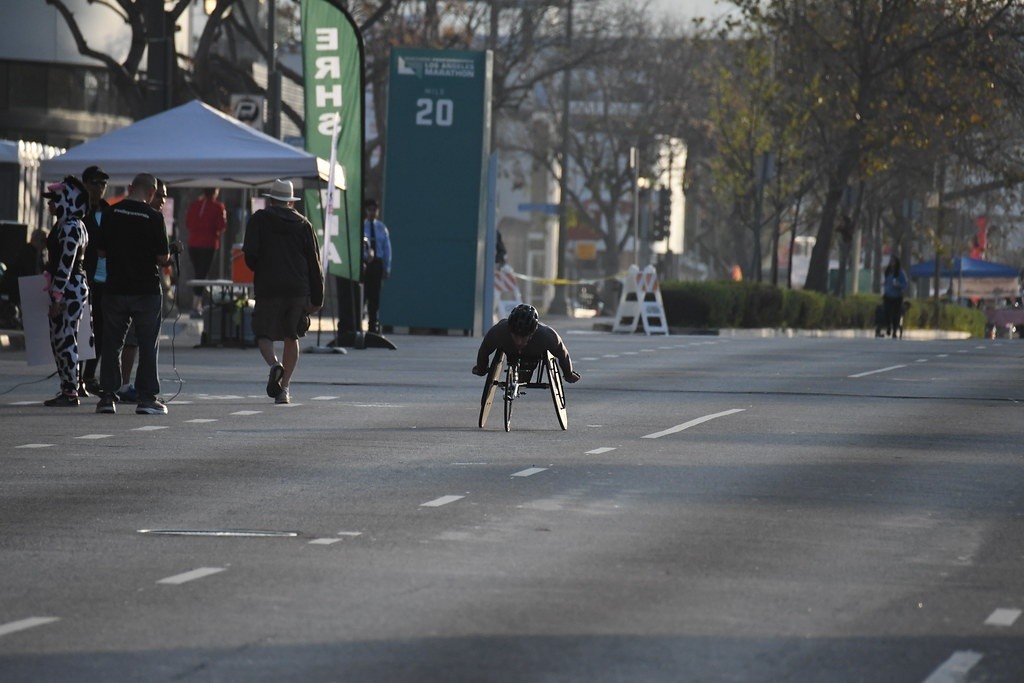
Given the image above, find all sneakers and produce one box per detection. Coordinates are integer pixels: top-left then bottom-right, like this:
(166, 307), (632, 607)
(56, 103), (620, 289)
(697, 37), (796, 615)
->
(56, 383), (89, 397)
(96, 397), (116, 413)
(83, 377), (101, 395)
(44, 395), (79, 406)
(136, 399), (168, 415)
(267, 362), (284, 398)
(275, 390), (289, 403)
(118, 384), (142, 401)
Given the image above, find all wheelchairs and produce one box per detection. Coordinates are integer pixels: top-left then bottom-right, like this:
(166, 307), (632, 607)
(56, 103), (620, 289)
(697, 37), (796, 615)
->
(479, 344), (568, 432)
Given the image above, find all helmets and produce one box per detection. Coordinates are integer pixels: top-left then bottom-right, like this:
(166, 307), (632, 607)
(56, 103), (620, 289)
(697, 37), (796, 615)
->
(507, 303), (538, 333)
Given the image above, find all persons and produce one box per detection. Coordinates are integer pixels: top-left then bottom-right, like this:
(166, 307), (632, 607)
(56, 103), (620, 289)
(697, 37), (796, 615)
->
(363, 199), (392, 335)
(24, 166), (168, 407)
(874, 254), (911, 341)
(244, 178), (325, 404)
(186, 187), (228, 319)
(95, 173), (184, 415)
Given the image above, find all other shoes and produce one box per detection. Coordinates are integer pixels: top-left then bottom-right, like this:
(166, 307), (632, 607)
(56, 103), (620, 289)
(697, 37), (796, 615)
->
(189, 308), (203, 317)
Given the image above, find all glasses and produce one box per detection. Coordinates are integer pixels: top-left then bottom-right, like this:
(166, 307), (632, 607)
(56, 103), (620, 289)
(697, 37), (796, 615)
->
(156, 194), (167, 200)
(89, 181), (107, 185)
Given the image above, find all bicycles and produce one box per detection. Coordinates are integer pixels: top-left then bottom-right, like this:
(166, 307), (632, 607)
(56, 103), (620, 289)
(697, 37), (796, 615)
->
(895, 295), (908, 337)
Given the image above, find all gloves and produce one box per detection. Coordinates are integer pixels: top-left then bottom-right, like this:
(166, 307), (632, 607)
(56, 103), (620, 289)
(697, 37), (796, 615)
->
(563, 371), (580, 383)
(472, 366), (490, 376)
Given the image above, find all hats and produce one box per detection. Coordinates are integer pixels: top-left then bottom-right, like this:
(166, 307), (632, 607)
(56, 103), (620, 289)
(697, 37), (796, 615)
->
(82, 166), (109, 181)
(41, 192), (53, 198)
(262, 178), (301, 201)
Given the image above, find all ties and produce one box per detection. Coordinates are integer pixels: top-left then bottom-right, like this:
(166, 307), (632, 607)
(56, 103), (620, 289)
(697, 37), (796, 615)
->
(369, 220), (376, 251)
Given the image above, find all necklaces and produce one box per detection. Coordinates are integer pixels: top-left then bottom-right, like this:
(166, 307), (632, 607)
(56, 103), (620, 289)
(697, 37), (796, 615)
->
(472, 304), (580, 384)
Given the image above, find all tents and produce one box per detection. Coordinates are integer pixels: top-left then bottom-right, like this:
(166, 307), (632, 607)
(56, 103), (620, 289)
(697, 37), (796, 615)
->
(910, 255), (1019, 307)
(37, 99), (398, 355)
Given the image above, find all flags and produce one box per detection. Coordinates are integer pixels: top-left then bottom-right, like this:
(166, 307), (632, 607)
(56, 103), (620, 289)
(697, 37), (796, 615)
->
(300, 0), (366, 282)
